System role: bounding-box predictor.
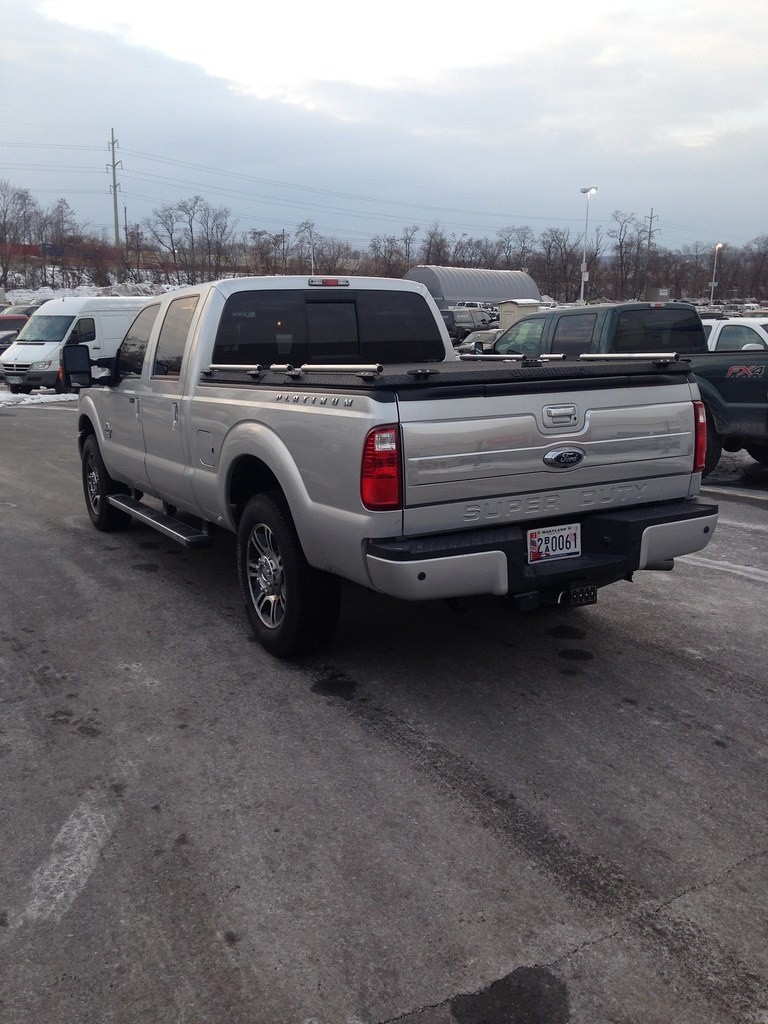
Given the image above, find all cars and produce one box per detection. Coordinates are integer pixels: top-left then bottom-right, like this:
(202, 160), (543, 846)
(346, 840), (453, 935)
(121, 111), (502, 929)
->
(0, 330), (19, 356)
(30, 298), (54, 307)
(0, 304), (42, 318)
(0, 313), (31, 334)
(439, 296), (768, 359)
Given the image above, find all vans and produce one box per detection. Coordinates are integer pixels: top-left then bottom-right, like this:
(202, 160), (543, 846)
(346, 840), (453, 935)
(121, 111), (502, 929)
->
(0, 295), (158, 395)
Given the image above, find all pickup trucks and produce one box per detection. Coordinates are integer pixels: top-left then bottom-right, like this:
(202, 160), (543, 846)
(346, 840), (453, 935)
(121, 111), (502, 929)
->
(463, 301), (768, 482)
(59, 274), (720, 661)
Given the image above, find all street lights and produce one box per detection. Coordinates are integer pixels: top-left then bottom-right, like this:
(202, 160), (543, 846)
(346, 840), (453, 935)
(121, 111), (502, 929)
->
(578, 187), (597, 305)
(710, 242), (724, 305)
